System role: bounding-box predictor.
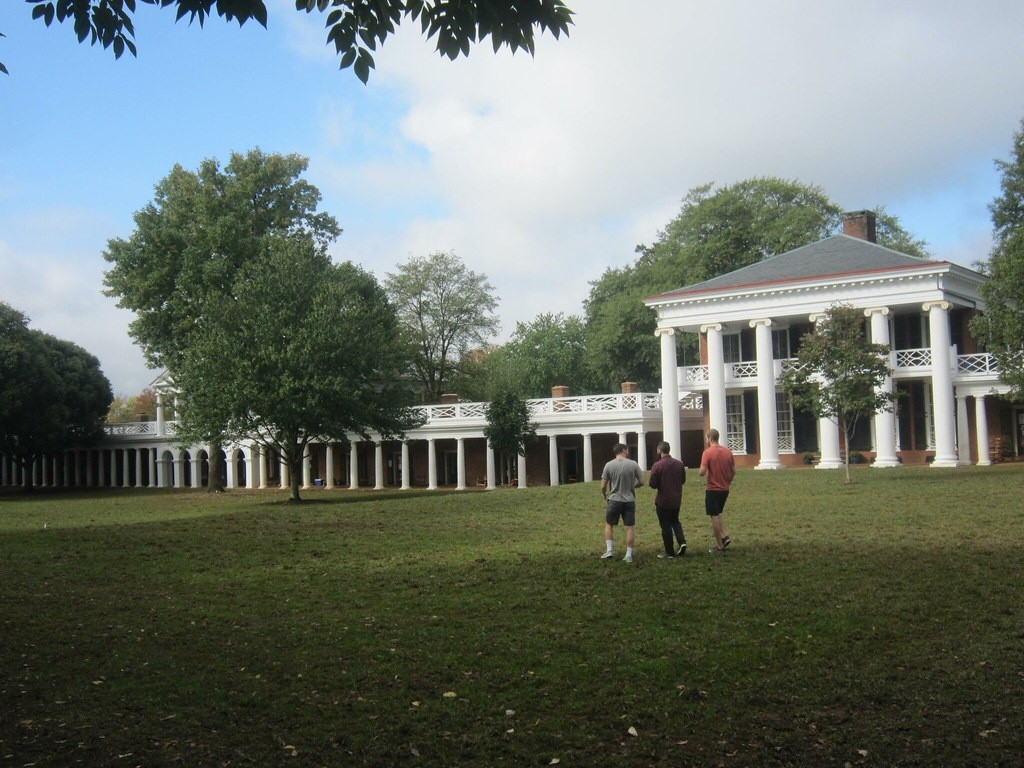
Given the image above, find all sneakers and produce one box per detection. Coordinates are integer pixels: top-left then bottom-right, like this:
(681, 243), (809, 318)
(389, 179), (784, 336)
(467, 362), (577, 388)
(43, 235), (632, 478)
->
(623, 555), (633, 562)
(600, 550), (613, 558)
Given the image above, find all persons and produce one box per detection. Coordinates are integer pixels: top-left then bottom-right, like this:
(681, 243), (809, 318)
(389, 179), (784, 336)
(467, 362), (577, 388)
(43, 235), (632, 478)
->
(601, 444), (644, 562)
(699, 429), (737, 553)
(649, 442), (688, 559)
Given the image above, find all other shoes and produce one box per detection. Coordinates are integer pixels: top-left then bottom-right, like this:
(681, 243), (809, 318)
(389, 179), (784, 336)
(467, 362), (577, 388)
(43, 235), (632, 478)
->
(722, 535), (730, 547)
(677, 542), (687, 556)
(709, 545), (724, 552)
(657, 553), (674, 559)
